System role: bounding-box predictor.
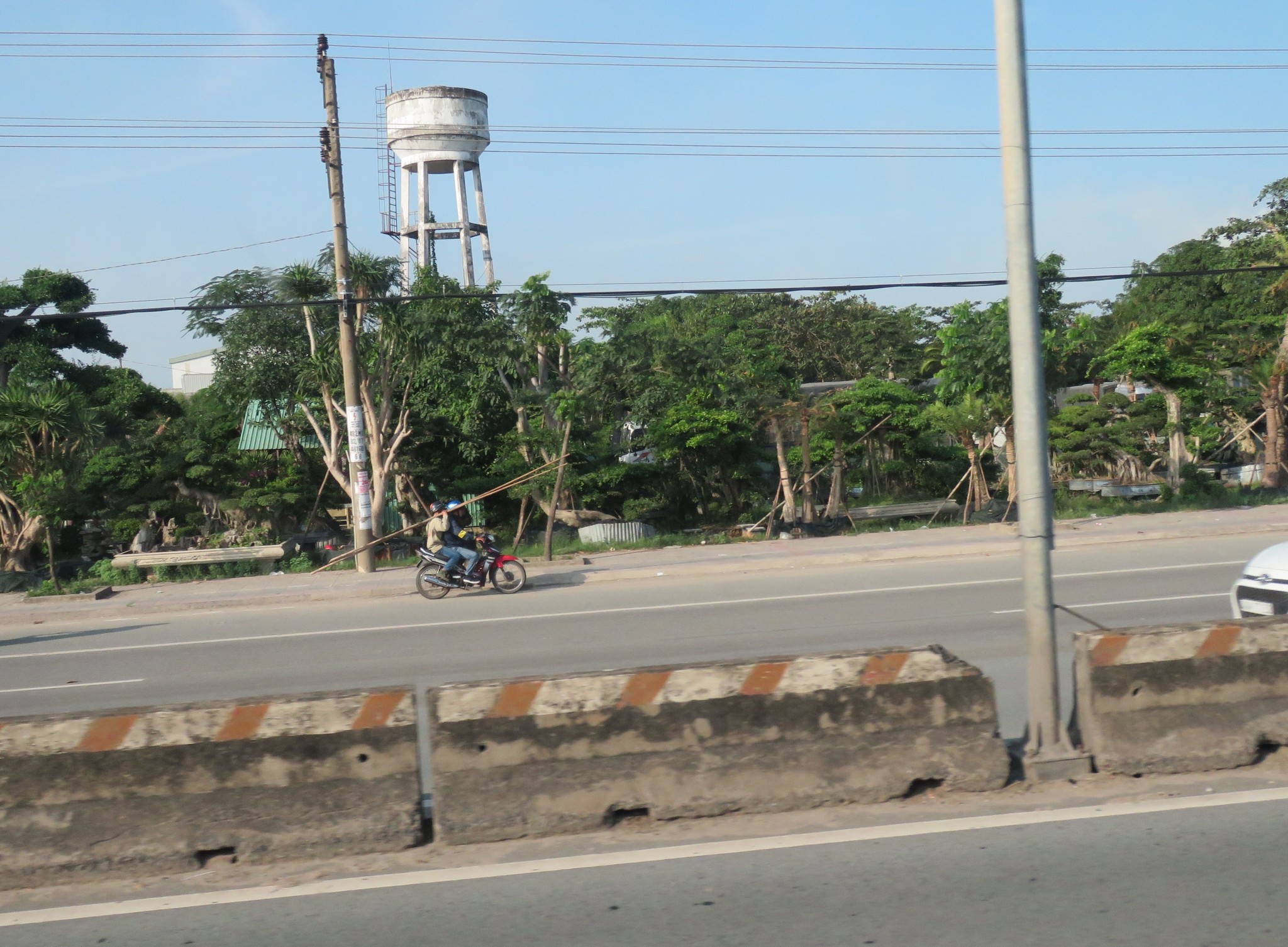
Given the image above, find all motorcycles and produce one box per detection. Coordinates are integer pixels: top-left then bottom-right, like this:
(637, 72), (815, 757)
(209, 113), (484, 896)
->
(415, 522), (526, 600)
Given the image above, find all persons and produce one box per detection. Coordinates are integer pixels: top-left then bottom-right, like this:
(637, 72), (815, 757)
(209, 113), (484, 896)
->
(425, 500), (461, 583)
(443, 501), (485, 584)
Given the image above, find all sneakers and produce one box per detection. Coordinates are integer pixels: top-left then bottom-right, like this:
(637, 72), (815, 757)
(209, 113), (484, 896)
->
(436, 569), (451, 583)
(463, 575), (480, 584)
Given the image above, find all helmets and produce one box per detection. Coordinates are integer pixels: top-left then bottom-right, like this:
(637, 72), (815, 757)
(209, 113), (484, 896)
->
(447, 500), (462, 511)
(430, 502), (445, 512)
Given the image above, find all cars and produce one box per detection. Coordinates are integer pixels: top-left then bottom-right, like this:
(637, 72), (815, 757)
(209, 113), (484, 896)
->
(1230, 541), (1288, 620)
